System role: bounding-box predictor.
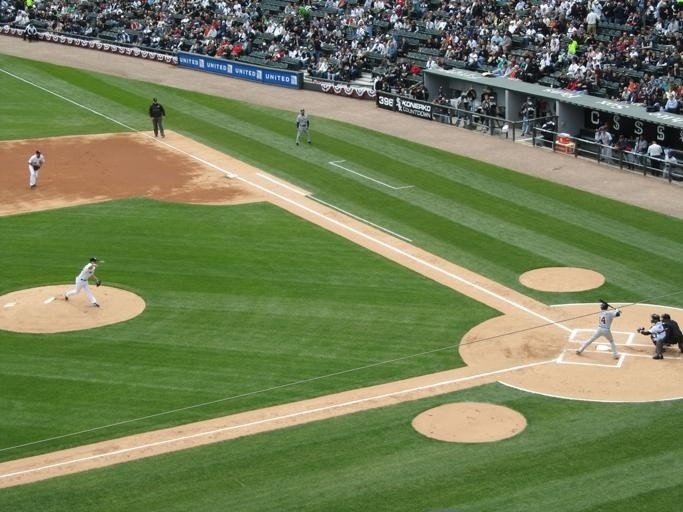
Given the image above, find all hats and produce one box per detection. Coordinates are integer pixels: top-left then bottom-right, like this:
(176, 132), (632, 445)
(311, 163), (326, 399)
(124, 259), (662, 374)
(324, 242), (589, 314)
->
(662, 314), (670, 319)
(36, 150), (40, 153)
(90, 258), (96, 261)
(153, 98), (157, 101)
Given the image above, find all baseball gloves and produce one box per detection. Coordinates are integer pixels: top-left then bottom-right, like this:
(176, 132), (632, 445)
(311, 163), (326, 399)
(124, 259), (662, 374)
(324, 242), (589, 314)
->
(637, 327), (645, 333)
(96, 280), (101, 286)
(34, 166), (39, 170)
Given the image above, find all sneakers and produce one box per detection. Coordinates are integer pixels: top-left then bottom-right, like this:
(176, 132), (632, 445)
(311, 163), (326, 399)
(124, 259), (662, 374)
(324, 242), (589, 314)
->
(93, 303), (99, 307)
(65, 292), (68, 300)
(652, 354), (662, 359)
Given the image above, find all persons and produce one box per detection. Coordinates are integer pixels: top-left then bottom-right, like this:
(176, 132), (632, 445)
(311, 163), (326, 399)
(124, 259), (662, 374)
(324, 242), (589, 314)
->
(28, 150), (44, 187)
(375, 1), (683, 179)
(295, 108), (311, 145)
(65, 258), (102, 307)
(653, 314), (683, 359)
(0, 1), (405, 83)
(575, 303), (622, 359)
(149, 97), (166, 138)
(637, 314), (667, 353)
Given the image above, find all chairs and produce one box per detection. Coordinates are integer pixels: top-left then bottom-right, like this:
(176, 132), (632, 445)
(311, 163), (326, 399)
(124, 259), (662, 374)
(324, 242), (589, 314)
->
(98, 0), (683, 96)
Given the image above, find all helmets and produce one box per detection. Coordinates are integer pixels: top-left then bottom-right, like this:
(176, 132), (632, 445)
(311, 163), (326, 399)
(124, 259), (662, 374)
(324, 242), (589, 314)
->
(601, 303), (608, 310)
(650, 314), (660, 323)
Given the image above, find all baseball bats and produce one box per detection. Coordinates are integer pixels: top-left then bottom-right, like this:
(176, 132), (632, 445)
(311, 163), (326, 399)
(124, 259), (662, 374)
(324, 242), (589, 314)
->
(599, 299), (622, 313)
